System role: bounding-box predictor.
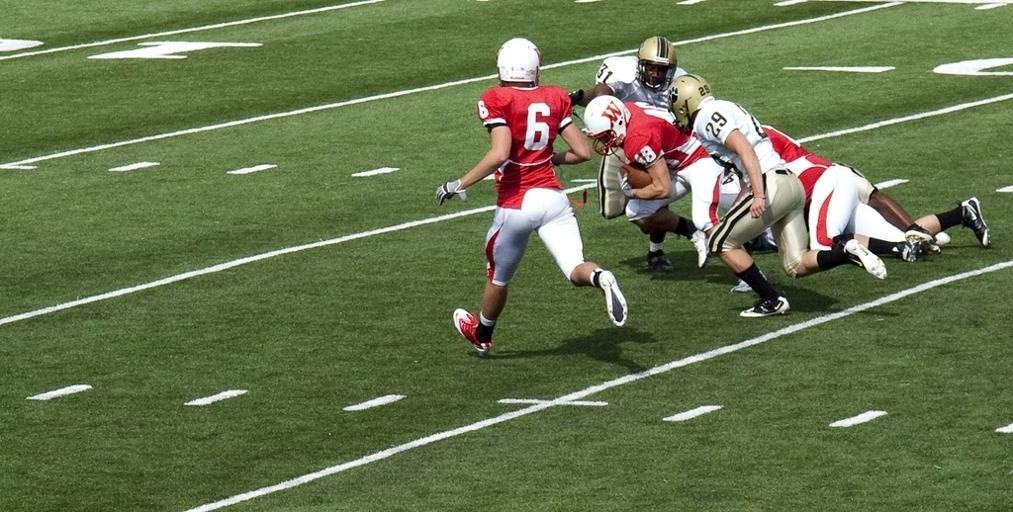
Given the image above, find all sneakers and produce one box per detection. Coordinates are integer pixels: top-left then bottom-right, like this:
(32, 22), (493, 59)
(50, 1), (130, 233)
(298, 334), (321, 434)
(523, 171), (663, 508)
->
(593, 270), (628, 327)
(843, 239), (887, 280)
(959, 197), (991, 247)
(892, 226), (951, 263)
(690, 229), (709, 269)
(731, 269), (791, 318)
(454, 308), (493, 352)
(647, 249), (673, 272)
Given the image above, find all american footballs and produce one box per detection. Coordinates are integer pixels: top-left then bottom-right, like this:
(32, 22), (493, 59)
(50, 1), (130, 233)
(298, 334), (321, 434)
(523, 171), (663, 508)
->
(620, 163), (652, 187)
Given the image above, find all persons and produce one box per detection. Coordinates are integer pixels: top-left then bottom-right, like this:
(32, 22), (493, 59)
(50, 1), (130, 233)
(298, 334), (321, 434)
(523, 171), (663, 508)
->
(761, 125), (991, 264)
(433, 35), (627, 357)
(582, 95), (775, 293)
(565, 37), (696, 272)
(665, 73), (886, 317)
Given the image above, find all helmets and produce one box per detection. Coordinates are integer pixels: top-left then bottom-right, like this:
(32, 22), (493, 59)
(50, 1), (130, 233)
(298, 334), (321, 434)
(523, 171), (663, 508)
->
(638, 36), (675, 91)
(497, 38), (542, 88)
(667, 74), (713, 128)
(582, 96), (632, 156)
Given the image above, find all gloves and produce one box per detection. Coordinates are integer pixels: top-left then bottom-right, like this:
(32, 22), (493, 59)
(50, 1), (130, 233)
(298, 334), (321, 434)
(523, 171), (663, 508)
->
(618, 172), (635, 198)
(435, 180), (467, 206)
